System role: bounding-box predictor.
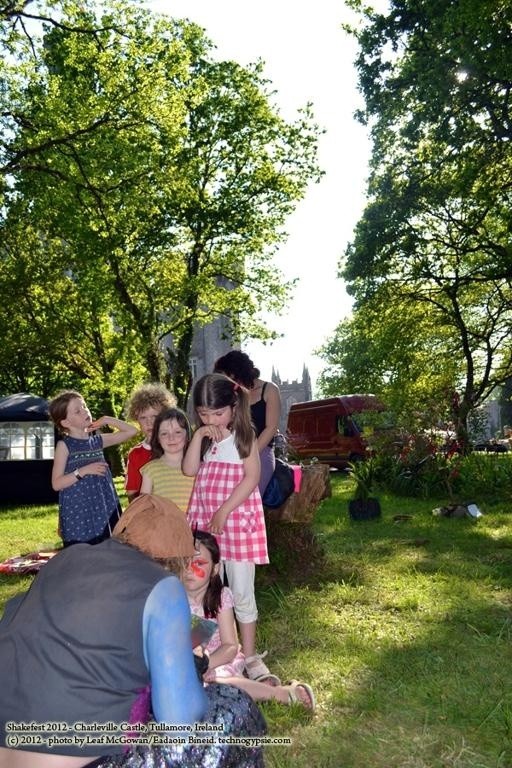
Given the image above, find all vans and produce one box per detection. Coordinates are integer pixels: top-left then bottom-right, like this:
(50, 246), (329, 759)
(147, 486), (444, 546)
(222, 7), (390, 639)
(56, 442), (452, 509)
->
(284, 395), (458, 470)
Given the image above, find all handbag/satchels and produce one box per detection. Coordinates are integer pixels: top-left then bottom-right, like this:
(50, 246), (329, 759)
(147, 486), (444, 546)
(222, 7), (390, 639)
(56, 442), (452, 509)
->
(442, 504), (465, 517)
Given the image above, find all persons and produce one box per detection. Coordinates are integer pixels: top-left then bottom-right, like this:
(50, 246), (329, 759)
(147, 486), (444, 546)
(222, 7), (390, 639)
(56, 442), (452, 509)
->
(174, 525), (317, 711)
(138, 405), (200, 515)
(0, 493), (266, 766)
(122, 381), (177, 508)
(49, 389), (139, 546)
(181, 372), (273, 684)
(210, 351), (284, 500)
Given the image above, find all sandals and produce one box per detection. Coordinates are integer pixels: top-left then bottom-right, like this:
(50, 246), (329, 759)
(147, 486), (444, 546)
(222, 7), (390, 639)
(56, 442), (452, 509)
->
(244, 656), (282, 687)
(287, 679), (315, 711)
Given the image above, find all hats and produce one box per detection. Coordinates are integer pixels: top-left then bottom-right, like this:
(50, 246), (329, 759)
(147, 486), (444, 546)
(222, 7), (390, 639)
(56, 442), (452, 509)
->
(112, 493), (201, 559)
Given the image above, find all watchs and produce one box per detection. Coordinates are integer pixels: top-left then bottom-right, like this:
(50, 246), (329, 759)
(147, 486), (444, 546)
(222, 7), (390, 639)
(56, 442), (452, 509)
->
(74, 467), (84, 483)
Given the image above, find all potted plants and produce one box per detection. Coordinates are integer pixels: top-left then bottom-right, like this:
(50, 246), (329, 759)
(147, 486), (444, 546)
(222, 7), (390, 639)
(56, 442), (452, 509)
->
(345, 459), (382, 522)
